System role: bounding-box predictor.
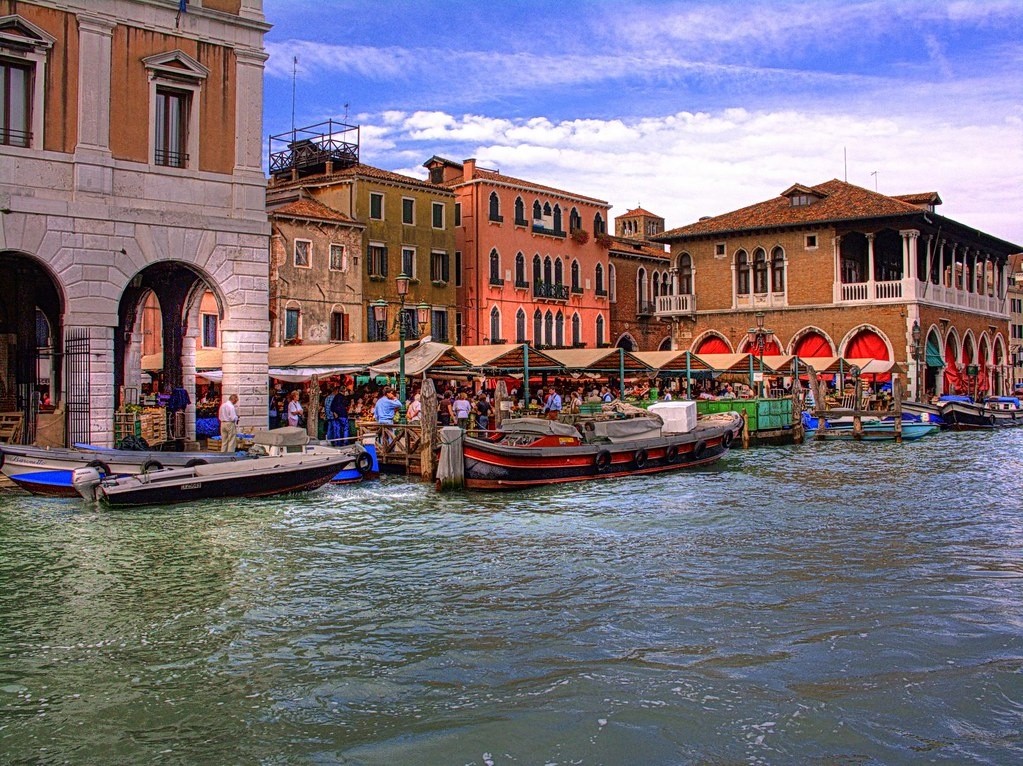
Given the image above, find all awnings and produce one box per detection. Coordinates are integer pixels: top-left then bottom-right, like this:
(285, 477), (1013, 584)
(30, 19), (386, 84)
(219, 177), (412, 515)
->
(926, 339), (946, 368)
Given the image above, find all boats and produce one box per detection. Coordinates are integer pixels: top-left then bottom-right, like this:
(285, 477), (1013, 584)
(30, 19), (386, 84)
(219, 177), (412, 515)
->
(0, 432), (378, 496)
(72, 426), (372, 512)
(434, 411), (745, 492)
(889, 396), (1023, 429)
(803, 413), (941, 441)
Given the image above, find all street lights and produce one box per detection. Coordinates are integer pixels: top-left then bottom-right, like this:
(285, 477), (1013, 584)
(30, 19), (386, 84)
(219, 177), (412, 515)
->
(368, 271), (432, 433)
(747, 312), (774, 397)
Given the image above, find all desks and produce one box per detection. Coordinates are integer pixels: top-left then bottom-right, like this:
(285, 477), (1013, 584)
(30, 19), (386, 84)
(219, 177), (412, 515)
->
(357, 422), (378, 440)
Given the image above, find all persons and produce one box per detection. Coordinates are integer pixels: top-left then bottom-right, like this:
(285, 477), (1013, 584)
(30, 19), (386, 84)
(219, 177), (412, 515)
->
(287, 390), (303, 426)
(473, 393), (494, 436)
(324, 386), (337, 445)
(453, 392), (471, 425)
(330, 384), (350, 445)
(407, 393), (421, 419)
(543, 386), (563, 420)
(218, 394), (241, 453)
(925, 384), (936, 401)
(976, 391), (984, 403)
(42, 392), (50, 405)
(439, 390), (458, 426)
(374, 385), (401, 444)
(198, 375), (891, 419)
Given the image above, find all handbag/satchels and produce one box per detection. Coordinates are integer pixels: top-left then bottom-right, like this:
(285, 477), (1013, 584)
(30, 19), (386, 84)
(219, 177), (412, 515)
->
(269, 409), (277, 418)
(298, 416), (305, 426)
(545, 408), (550, 413)
(573, 400), (580, 414)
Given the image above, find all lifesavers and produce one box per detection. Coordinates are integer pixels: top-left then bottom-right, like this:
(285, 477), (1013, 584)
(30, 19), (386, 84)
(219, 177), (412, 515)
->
(978, 407), (985, 417)
(355, 452), (372, 474)
(988, 414), (996, 425)
(633, 449), (648, 469)
(593, 449), (611, 472)
(184, 458), (209, 468)
(142, 459), (163, 474)
(722, 429), (734, 449)
(85, 459), (111, 477)
(666, 445), (678, 463)
(692, 440), (706, 459)
(1010, 412), (1016, 420)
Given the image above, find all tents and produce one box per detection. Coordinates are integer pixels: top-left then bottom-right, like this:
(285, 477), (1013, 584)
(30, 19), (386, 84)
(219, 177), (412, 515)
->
(141, 340), (902, 382)
